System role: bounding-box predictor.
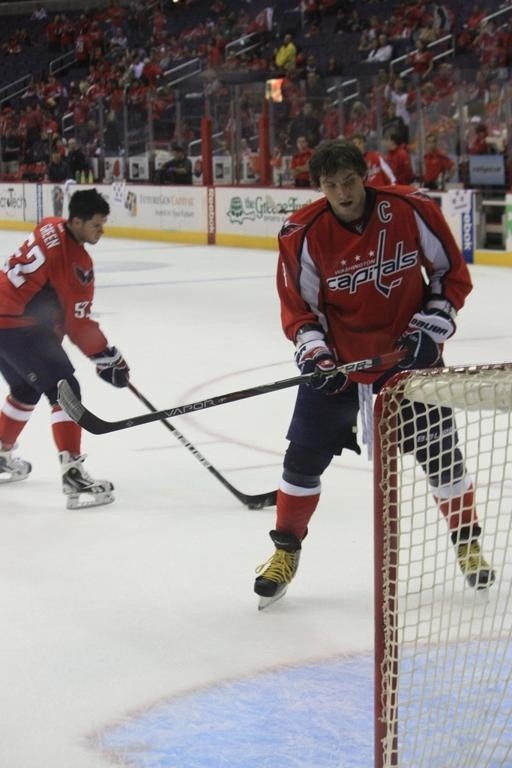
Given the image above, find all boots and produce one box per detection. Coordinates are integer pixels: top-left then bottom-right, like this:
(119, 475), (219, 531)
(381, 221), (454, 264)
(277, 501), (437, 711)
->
(451, 525), (494, 589)
(254, 526), (308, 597)
(0, 454), (31, 474)
(59, 449), (115, 493)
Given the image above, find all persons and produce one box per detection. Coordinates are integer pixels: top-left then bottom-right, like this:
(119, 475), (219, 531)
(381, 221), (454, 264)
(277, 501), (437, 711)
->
(0, 189), (129, 494)
(2, 1), (511, 190)
(252, 136), (496, 598)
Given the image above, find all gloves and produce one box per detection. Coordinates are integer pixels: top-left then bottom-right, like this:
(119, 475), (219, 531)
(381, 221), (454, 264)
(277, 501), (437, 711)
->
(395, 313), (457, 370)
(295, 339), (349, 395)
(93, 346), (130, 387)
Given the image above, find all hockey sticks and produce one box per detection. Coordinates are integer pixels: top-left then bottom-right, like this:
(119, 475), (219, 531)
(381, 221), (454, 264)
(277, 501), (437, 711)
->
(126, 380), (278, 510)
(58, 348), (409, 435)
